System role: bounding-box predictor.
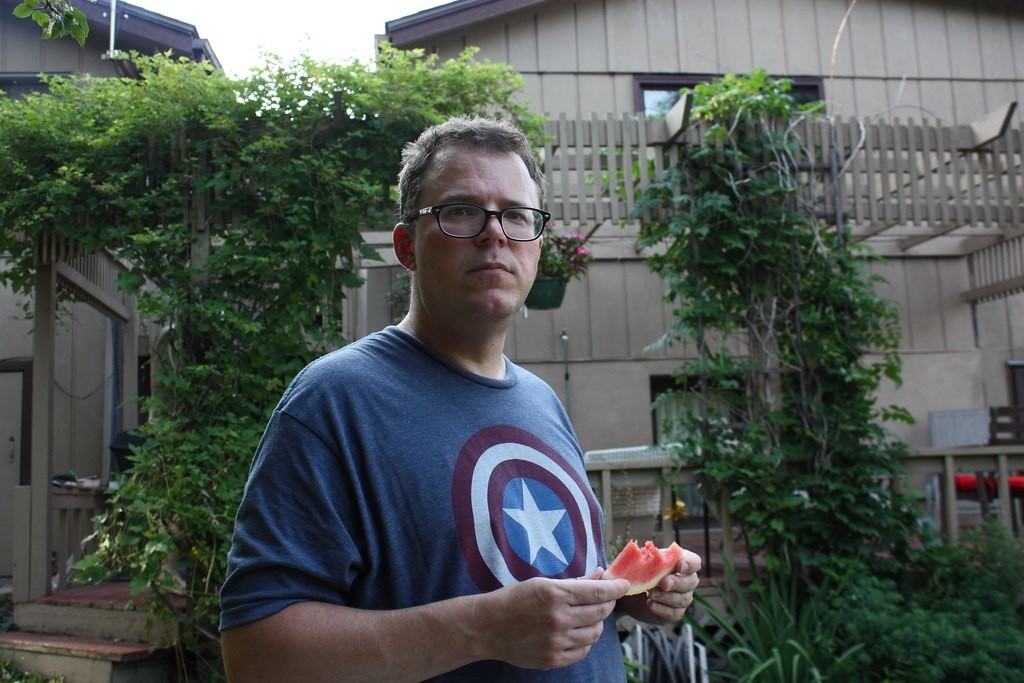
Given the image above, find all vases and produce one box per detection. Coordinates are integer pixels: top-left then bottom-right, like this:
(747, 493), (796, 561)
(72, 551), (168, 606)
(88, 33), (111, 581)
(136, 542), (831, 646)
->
(525, 278), (569, 310)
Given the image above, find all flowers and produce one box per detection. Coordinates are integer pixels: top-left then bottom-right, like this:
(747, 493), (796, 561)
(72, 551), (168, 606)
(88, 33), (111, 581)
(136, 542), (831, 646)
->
(537, 221), (595, 282)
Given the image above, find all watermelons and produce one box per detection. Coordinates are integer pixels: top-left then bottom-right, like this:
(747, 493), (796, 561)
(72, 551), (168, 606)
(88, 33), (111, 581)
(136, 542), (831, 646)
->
(599, 539), (685, 596)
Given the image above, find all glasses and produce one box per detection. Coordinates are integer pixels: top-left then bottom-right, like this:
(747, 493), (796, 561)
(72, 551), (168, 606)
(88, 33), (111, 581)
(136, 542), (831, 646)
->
(407, 204), (551, 242)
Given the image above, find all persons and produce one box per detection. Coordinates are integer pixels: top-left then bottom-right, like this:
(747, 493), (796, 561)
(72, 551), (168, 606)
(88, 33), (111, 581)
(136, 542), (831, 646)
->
(220, 113), (701, 683)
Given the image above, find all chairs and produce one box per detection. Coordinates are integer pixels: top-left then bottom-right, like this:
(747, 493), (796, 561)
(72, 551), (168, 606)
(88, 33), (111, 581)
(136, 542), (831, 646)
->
(925, 407), (1024, 547)
